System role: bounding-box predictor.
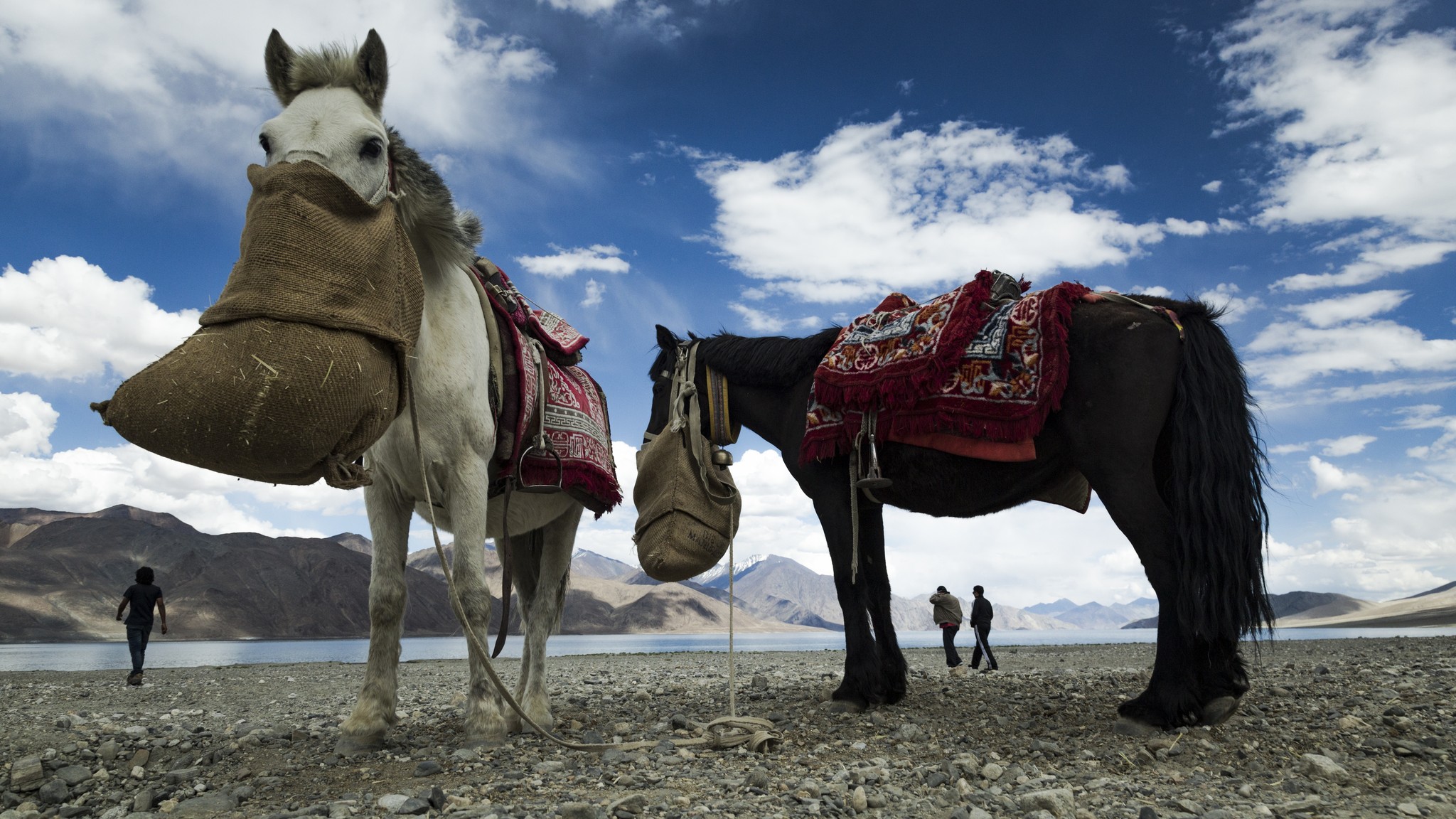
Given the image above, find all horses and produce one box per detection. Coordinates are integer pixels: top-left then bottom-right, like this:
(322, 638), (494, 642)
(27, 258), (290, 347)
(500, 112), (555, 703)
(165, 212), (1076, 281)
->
(258, 23), (587, 759)
(645, 266), (1275, 738)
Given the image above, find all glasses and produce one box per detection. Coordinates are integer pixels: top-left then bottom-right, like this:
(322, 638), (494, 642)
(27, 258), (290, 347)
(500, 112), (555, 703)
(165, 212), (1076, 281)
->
(972, 591), (976, 594)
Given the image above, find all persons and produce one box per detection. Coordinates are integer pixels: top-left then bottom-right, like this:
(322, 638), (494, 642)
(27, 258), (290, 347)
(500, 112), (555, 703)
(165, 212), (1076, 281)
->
(929, 586), (963, 668)
(968, 586), (998, 670)
(115, 566), (168, 684)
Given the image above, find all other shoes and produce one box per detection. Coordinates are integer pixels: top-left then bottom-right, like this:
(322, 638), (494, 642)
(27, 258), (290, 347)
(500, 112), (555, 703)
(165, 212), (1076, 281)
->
(968, 664), (978, 669)
(957, 659), (963, 666)
(984, 668), (991, 670)
(127, 672), (143, 685)
